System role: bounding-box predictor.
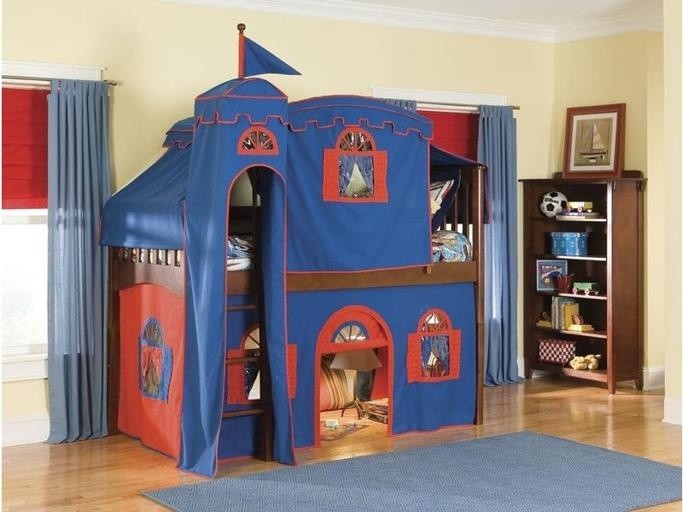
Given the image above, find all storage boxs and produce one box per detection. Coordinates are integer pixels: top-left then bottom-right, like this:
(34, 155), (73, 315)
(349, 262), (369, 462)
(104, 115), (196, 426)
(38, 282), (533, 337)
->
(537, 336), (577, 368)
(549, 230), (588, 258)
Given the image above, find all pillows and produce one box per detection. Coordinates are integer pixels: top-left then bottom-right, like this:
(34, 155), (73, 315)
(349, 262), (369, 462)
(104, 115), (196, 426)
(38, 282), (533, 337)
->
(430, 166), (462, 231)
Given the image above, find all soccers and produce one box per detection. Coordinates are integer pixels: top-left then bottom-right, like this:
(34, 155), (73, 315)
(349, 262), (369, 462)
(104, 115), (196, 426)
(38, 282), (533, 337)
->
(539, 191), (567, 218)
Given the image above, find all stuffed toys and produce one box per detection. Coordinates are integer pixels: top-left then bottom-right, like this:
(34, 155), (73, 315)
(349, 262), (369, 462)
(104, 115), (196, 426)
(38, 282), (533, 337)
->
(568, 354), (601, 371)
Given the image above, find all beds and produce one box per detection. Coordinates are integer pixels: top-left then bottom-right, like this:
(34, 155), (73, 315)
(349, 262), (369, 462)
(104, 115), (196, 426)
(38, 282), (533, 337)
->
(101, 160), (490, 474)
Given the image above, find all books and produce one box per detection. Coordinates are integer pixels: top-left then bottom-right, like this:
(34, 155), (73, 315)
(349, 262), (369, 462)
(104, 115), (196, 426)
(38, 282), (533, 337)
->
(555, 211), (604, 220)
(535, 295), (595, 332)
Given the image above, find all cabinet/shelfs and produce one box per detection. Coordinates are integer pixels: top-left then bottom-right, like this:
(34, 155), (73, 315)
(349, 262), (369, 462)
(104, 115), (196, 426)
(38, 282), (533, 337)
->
(515, 169), (645, 394)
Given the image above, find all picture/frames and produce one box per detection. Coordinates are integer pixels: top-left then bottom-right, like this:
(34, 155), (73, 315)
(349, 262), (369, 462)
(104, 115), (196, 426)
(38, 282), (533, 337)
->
(535, 258), (569, 291)
(561, 102), (627, 181)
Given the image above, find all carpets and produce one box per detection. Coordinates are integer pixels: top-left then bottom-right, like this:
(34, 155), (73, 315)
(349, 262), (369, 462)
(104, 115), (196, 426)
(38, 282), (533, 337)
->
(138, 429), (681, 512)
